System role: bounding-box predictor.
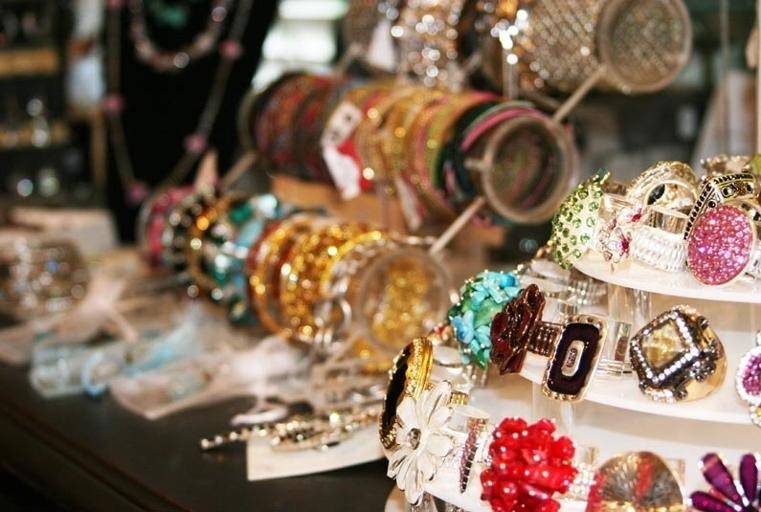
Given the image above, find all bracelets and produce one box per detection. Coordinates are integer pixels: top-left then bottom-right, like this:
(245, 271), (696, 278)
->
(340, 0), (696, 98)
(254, 67), (579, 225)
(129, 178), (453, 367)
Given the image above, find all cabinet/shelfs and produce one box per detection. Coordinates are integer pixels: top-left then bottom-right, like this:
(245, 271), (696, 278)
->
(381, 254), (760, 511)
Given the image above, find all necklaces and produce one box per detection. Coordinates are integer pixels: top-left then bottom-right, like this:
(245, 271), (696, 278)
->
(106, 0), (252, 206)
(125, 0), (230, 75)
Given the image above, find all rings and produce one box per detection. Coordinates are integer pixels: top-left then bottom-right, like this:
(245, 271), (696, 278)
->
(547, 141), (761, 293)
(378, 334), (760, 512)
(453, 267), (760, 420)
